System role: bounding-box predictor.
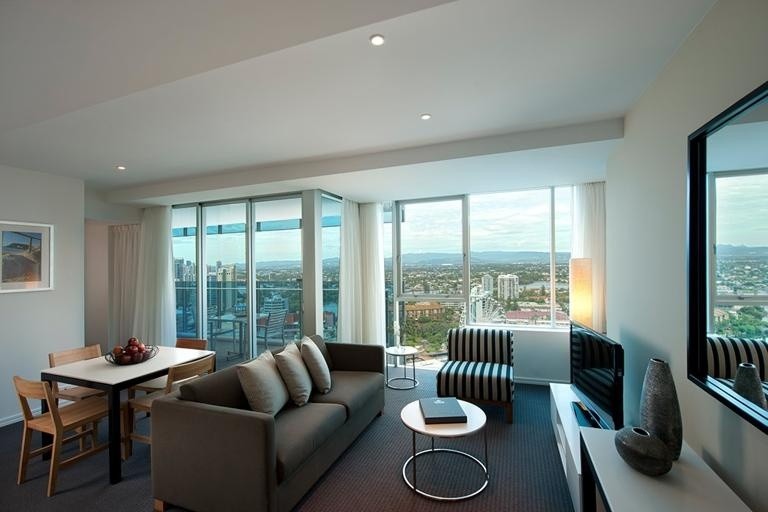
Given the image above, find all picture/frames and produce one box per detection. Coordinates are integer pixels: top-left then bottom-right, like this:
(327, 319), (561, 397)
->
(0, 221), (54, 294)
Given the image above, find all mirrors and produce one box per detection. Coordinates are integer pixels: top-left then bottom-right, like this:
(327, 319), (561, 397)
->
(683, 78), (766, 434)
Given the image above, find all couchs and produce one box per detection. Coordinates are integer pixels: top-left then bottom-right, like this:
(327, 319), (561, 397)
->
(711, 335), (766, 391)
(150, 334), (384, 511)
(436, 326), (514, 426)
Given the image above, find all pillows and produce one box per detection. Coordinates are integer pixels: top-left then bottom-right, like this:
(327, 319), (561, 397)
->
(300, 335), (332, 394)
(235, 349), (288, 418)
(274, 341), (312, 405)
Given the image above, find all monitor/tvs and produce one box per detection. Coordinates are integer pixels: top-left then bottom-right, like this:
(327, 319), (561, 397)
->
(569, 320), (624, 431)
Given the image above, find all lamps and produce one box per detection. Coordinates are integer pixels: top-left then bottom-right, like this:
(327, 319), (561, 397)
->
(569, 256), (594, 330)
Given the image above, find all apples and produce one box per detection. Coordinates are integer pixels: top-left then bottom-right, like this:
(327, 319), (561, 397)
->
(113, 337), (145, 365)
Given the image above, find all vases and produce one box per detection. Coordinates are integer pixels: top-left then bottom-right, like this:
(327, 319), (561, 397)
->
(613, 426), (674, 482)
(732, 362), (763, 411)
(637, 357), (684, 464)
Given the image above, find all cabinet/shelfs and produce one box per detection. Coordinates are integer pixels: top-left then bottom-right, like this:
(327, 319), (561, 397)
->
(547, 381), (644, 512)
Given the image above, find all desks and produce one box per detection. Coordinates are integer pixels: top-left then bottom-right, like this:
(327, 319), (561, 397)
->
(209, 311), (269, 362)
(577, 423), (754, 512)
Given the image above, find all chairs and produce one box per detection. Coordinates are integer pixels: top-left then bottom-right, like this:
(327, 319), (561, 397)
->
(127, 337), (208, 418)
(125, 354), (215, 461)
(13, 375), (110, 498)
(254, 309), (287, 351)
(45, 343), (106, 452)
